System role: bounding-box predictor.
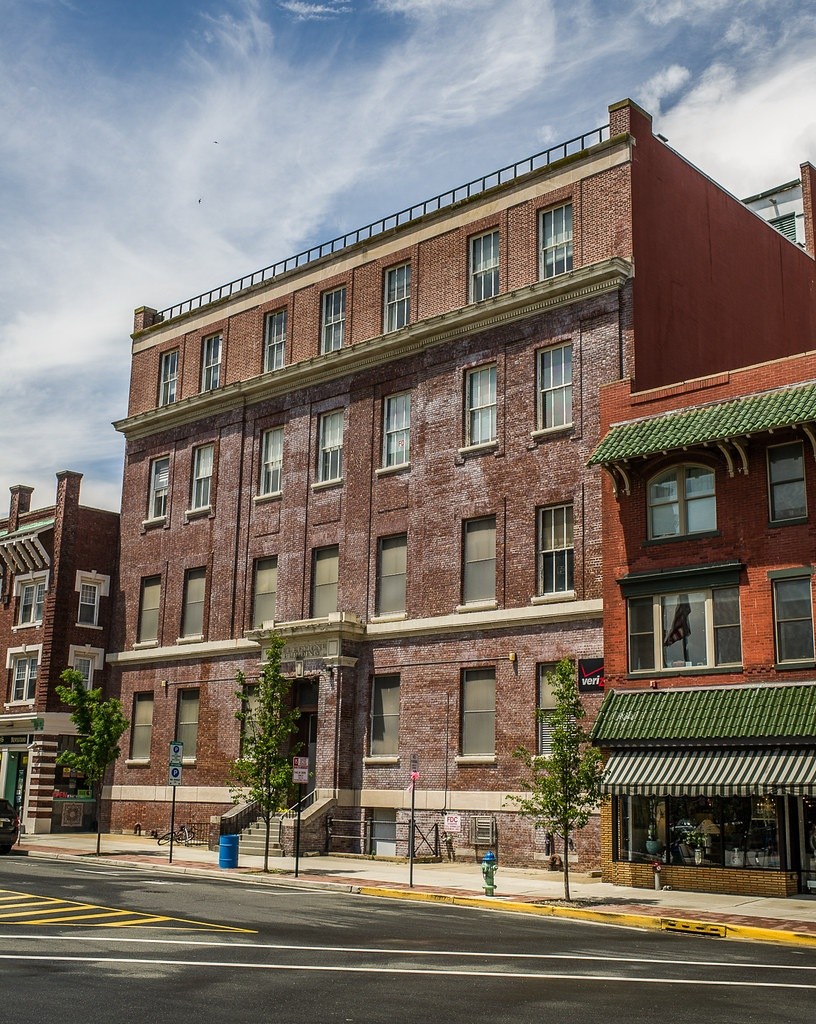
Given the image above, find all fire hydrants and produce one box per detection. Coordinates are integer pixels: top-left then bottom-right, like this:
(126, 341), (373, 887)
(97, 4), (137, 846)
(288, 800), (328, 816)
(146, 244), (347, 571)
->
(481, 852), (498, 896)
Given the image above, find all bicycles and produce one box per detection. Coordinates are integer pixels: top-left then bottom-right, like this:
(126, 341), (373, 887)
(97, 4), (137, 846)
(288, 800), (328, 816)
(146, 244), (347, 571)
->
(157, 825), (197, 846)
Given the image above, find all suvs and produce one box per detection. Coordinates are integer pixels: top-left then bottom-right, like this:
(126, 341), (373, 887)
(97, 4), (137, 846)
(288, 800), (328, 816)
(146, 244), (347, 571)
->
(0, 797), (20, 853)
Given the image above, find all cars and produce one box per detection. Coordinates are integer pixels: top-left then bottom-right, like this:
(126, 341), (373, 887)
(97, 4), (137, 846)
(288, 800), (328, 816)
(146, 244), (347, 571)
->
(729, 842), (780, 868)
